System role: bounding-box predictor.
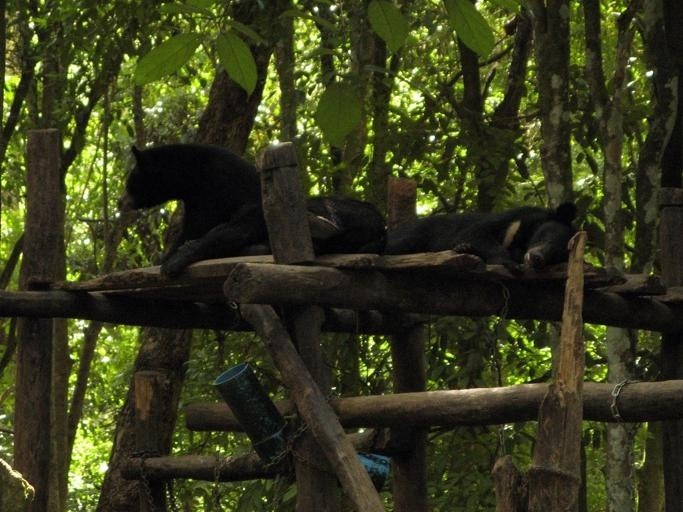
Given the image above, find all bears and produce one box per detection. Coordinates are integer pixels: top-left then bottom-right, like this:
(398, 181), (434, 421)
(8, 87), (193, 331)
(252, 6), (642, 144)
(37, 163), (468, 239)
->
(118, 142), (386, 284)
(384, 200), (580, 275)
